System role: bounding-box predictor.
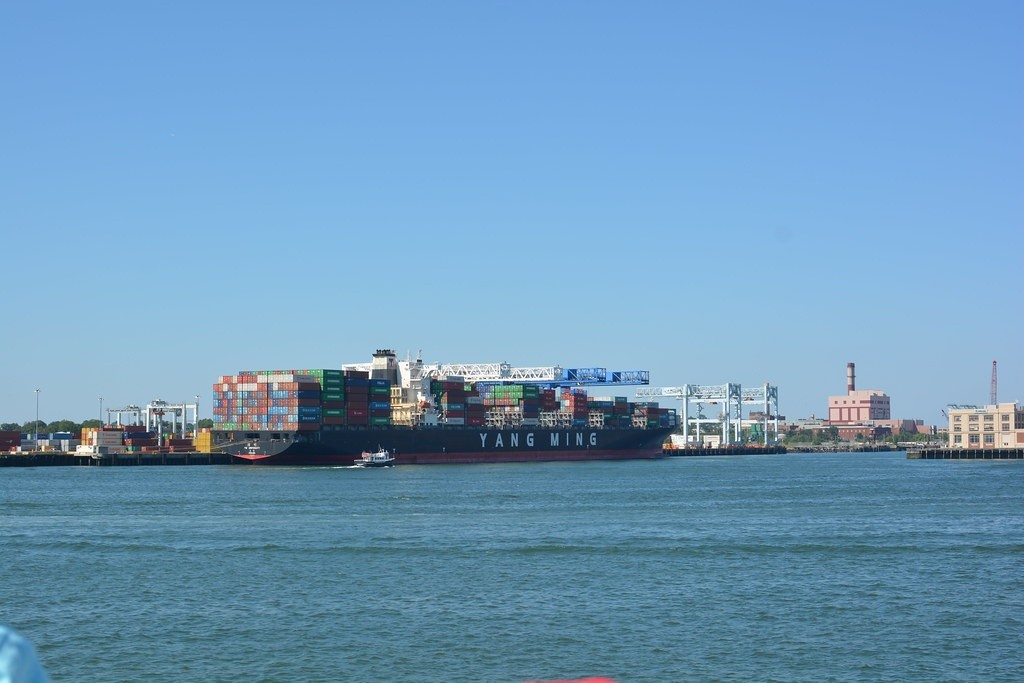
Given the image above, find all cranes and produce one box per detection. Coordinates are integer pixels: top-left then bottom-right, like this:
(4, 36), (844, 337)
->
(991, 359), (999, 406)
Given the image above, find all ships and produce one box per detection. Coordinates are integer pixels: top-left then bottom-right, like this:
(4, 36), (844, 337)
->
(209, 365), (684, 464)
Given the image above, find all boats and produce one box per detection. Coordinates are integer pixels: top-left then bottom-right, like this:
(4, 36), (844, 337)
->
(354, 443), (398, 469)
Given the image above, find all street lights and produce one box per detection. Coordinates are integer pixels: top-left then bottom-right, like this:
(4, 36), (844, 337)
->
(97, 396), (105, 428)
(34, 388), (42, 451)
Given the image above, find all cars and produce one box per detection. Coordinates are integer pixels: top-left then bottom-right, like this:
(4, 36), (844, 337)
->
(51, 447), (62, 451)
